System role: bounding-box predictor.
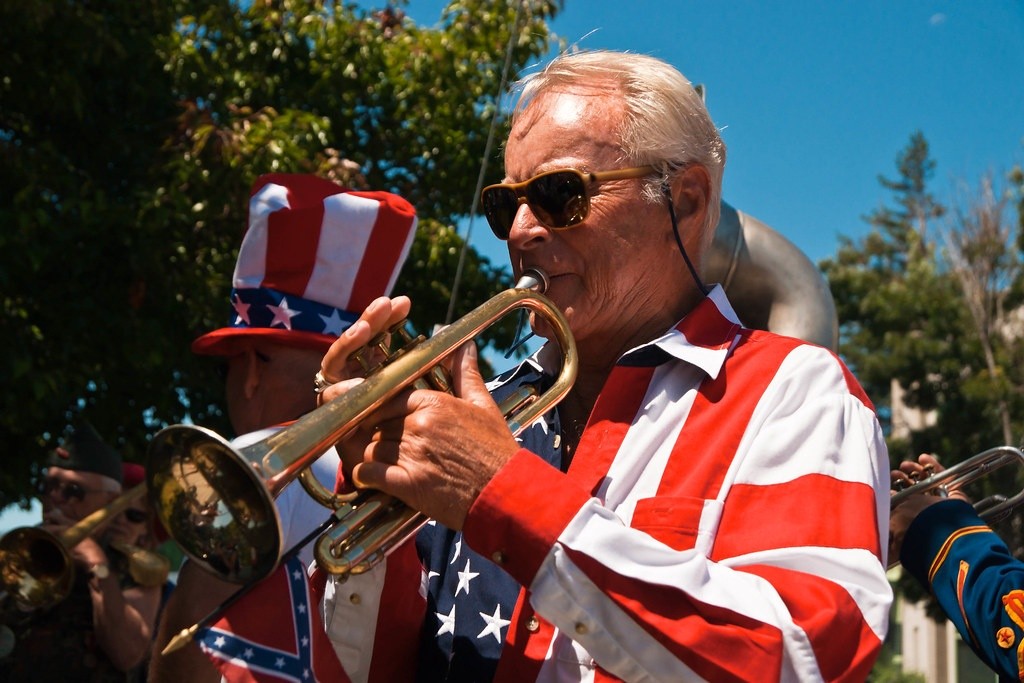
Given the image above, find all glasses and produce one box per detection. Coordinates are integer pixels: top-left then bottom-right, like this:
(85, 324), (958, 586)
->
(124, 506), (153, 523)
(478, 161), (683, 239)
(41, 476), (110, 504)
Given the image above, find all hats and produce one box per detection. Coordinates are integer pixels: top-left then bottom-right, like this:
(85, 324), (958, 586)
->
(119, 463), (146, 486)
(30, 414), (120, 482)
(189, 172), (419, 352)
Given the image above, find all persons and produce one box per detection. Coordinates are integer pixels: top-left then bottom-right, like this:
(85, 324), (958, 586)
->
(146, 171), (427, 683)
(890, 453), (1023, 682)
(312, 49), (890, 683)
(2, 442), (177, 683)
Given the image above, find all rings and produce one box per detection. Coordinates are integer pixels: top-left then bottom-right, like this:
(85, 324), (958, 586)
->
(313, 369), (332, 394)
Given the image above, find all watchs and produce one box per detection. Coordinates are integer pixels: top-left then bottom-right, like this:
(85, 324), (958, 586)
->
(87, 564), (112, 579)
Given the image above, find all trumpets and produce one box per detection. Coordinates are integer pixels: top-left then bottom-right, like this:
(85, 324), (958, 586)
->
(143, 266), (578, 659)
(887, 445), (1024, 572)
(2, 477), (149, 615)
